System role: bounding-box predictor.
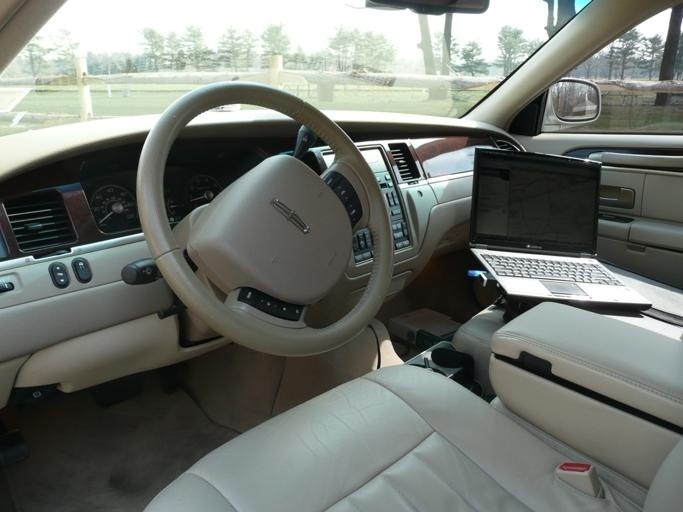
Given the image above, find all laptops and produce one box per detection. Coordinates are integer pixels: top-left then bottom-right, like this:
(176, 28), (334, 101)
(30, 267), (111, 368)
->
(467, 147), (652, 311)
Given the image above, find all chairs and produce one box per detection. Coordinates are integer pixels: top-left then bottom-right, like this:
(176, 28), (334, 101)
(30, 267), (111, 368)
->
(454, 256), (682, 360)
(136, 362), (683, 512)
